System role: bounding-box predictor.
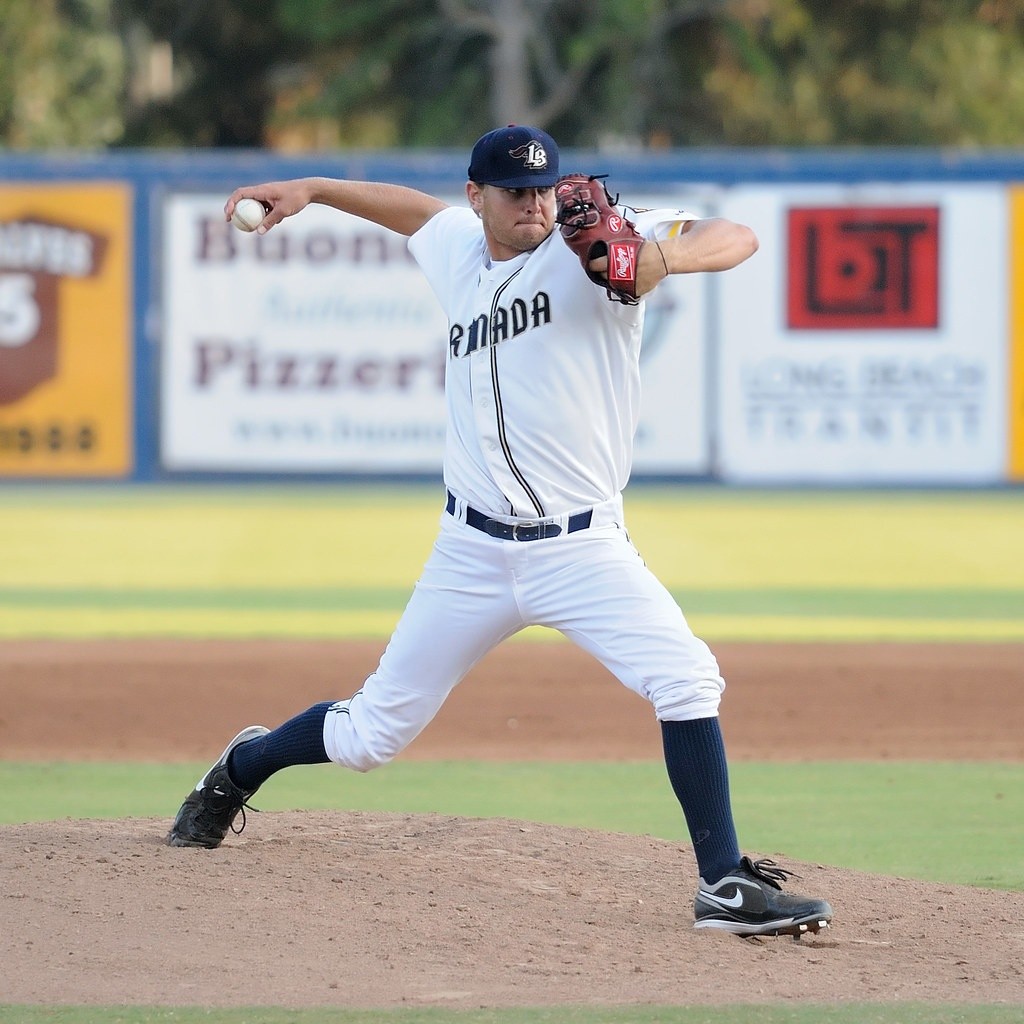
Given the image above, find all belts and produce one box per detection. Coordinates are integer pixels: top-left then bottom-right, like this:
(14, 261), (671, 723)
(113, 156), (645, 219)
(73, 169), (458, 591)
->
(445, 490), (593, 542)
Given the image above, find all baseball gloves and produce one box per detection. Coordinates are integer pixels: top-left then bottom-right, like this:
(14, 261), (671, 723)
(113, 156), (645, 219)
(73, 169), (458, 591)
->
(553, 172), (646, 305)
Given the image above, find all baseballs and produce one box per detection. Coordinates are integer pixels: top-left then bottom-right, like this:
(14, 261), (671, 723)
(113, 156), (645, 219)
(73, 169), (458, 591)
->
(230, 197), (267, 232)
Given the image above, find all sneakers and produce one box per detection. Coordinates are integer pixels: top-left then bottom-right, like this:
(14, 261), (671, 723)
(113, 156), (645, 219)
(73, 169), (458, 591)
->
(693, 856), (833, 937)
(169, 726), (271, 848)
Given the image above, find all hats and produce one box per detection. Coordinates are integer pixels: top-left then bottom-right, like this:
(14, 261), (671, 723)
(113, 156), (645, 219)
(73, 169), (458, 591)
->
(468, 123), (560, 189)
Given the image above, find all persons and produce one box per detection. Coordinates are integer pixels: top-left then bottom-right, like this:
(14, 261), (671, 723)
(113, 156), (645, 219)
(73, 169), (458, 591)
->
(165, 124), (834, 942)
